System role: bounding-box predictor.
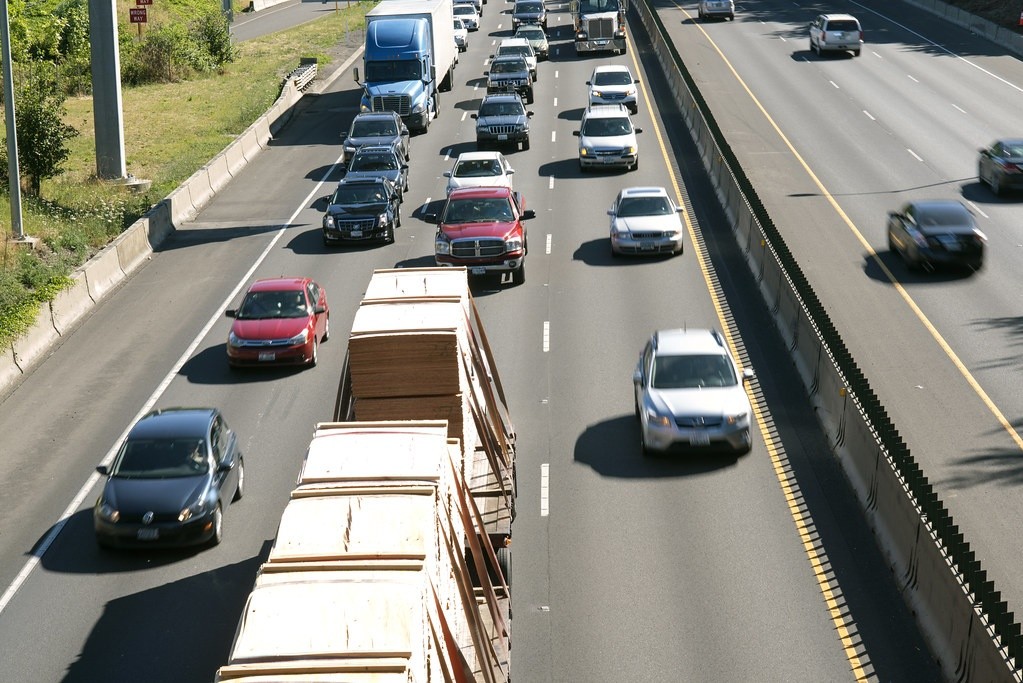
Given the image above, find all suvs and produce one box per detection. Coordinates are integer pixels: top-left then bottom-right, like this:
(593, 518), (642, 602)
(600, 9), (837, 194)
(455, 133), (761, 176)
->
(424, 187), (536, 283)
(585, 64), (641, 114)
(632, 327), (755, 464)
(573, 105), (643, 172)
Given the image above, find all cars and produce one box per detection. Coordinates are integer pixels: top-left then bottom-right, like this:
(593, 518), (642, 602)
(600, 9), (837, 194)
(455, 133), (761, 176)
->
(95, 406), (246, 556)
(223, 274), (332, 370)
(809, 13), (863, 56)
(698, 0), (736, 22)
(976, 137), (1023, 200)
(443, 1), (552, 195)
(887, 198), (985, 280)
(338, 111), (411, 170)
(320, 178), (402, 249)
(607, 186), (685, 257)
(338, 144), (409, 197)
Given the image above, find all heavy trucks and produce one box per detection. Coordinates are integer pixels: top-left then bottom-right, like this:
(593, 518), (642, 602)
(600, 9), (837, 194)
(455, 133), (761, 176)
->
(568, 1), (628, 55)
(353, 0), (456, 133)
(212, 266), (520, 683)
